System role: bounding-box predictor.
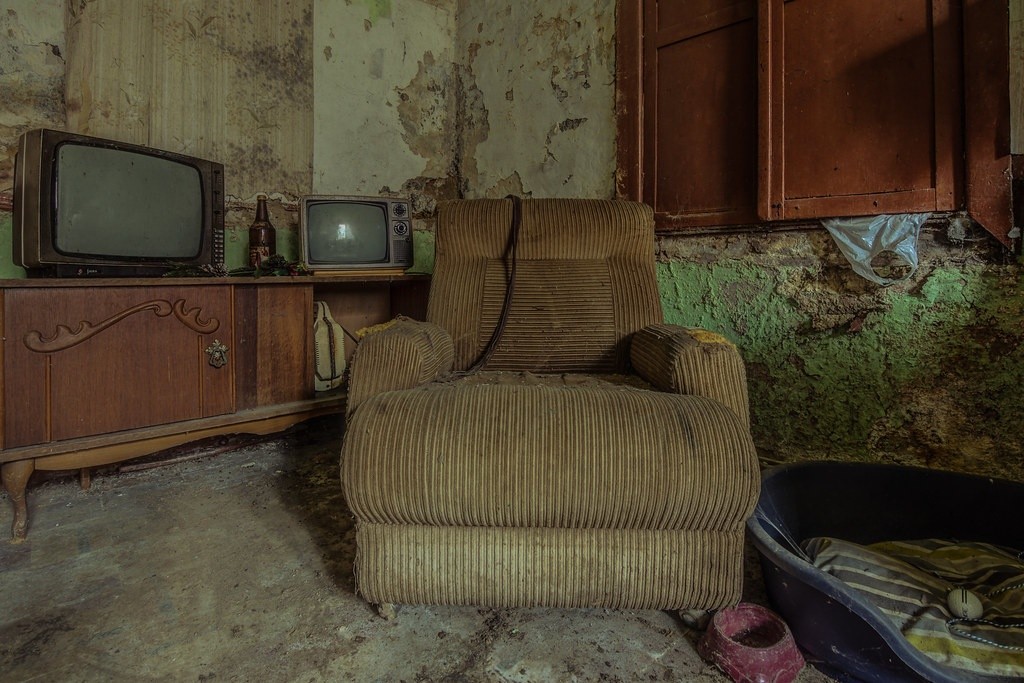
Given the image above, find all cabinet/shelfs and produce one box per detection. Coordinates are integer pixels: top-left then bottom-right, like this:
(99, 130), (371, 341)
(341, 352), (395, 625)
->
(0, 276), (434, 478)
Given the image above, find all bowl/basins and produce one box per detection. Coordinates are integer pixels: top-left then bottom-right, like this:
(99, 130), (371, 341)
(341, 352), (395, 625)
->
(698, 602), (805, 683)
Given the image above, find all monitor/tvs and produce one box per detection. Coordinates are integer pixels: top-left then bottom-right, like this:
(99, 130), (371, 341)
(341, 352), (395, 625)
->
(297, 194), (414, 271)
(12, 128), (224, 278)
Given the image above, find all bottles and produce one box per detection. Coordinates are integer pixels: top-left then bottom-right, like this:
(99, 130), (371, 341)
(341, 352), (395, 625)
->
(249, 194), (276, 269)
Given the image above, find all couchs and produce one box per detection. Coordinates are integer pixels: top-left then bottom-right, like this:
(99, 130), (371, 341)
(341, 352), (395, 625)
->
(339, 197), (761, 623)
